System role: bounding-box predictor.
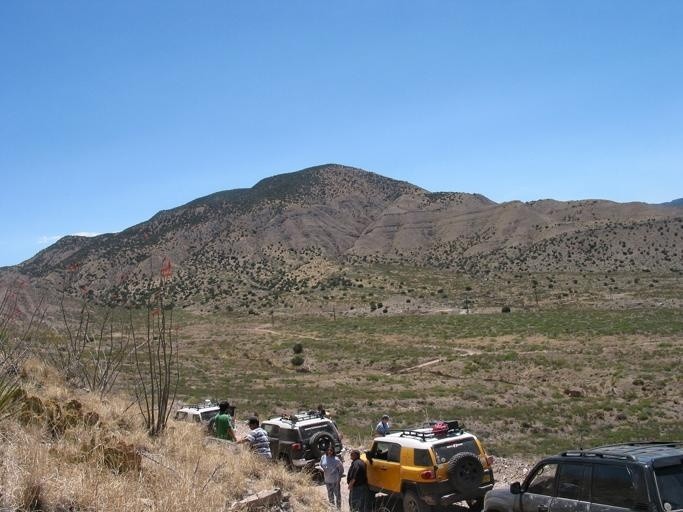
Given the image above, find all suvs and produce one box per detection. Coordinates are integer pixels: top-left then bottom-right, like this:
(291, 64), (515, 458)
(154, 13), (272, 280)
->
(484, 440), (682, 512)
(260, 410), (345, 471)
(175, 400), (220, 437)
(360, 419), (494, 511)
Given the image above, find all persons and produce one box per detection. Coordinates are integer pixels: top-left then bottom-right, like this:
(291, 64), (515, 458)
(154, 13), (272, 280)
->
(374, 415), (389, 435)
(235, 417), (271, 462)
(213, 400), (236, 443)
(346, 450), (369, 512)
(320, 445), (344, 509)
